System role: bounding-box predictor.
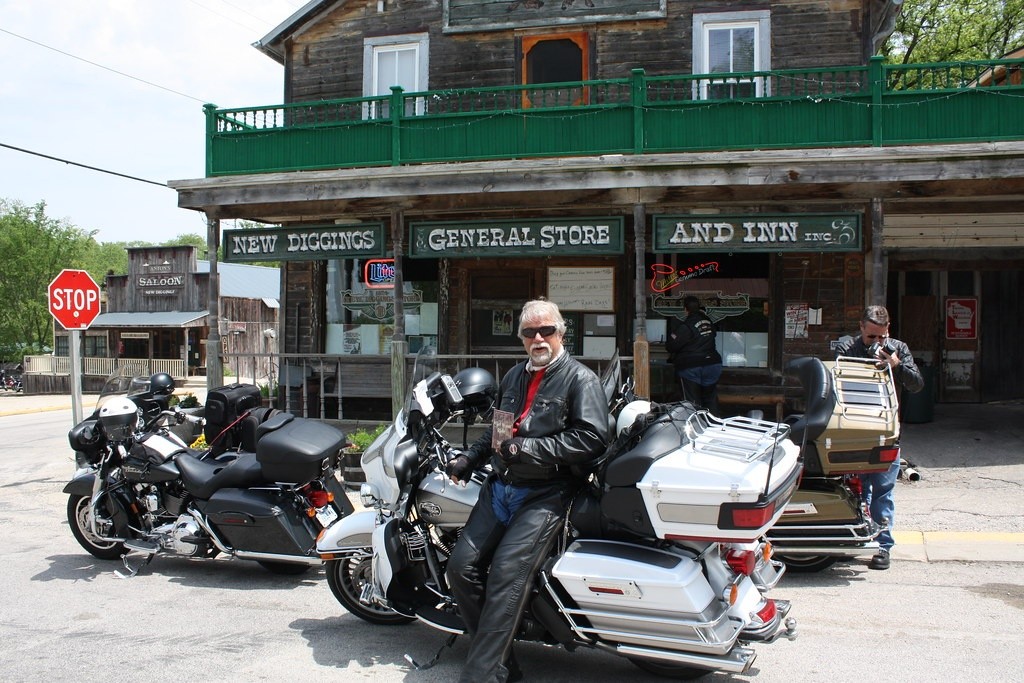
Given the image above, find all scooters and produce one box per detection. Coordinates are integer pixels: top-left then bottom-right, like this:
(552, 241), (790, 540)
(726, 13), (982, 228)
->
(0, 376), (24, 392)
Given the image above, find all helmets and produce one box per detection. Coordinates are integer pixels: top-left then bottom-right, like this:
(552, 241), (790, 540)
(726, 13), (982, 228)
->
(146, 372), (175, 395)
(453, 368), (498, 401)
(99, 397), (139, 441)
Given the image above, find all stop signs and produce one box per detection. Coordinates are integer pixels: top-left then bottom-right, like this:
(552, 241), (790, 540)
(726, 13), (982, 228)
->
(48, 269), (102, 331)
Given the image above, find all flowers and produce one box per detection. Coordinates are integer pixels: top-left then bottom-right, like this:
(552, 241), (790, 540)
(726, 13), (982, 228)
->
(346, 423), (387, 452)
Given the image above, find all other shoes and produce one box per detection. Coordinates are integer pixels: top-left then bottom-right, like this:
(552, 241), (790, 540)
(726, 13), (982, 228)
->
(504, 647), (523, 683)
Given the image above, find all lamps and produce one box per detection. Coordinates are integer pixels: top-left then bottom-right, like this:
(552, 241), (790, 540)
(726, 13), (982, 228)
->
(163, 261), (169, 264)
(142, 263), (149, 266)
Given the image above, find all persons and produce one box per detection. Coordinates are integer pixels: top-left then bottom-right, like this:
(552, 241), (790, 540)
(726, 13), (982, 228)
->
(445, 299), (614, 683)
(666, 296), (723, 422)
(836, 306), (925, 570)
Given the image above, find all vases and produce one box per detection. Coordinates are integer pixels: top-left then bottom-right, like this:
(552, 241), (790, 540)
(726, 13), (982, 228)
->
(343, 453), (362, 467)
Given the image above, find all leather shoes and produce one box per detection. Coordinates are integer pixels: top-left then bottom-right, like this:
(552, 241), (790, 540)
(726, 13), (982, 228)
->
(871, 549), (890, 568)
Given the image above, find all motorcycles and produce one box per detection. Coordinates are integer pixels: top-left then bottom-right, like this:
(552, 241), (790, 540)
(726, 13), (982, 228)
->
(568, 348), (900, 573)
(315, 347), (806, 683)
(60, 365), (353, 578)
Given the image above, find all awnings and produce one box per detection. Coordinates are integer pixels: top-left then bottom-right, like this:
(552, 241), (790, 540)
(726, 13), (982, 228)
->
(90, 312), (209, 327)
(262, 298), (280, 309)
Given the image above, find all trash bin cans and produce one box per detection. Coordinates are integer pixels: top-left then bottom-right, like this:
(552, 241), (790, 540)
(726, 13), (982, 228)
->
(901, 366), (937, 424)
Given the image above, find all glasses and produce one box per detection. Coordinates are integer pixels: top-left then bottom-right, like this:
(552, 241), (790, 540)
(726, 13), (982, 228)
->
(521, 326), (556, 338)
(864, 332), (888, 339)
